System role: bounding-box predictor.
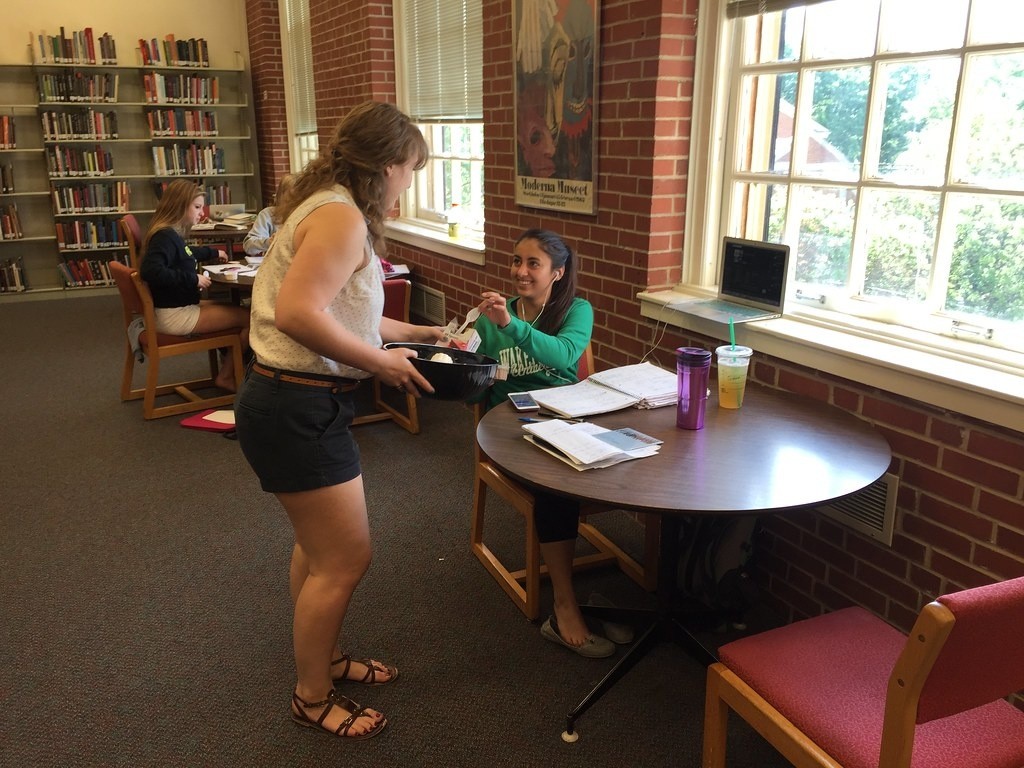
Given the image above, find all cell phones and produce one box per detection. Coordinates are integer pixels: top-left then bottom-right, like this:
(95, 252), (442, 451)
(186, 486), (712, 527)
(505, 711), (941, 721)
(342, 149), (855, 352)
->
(507, 392), (540, 412)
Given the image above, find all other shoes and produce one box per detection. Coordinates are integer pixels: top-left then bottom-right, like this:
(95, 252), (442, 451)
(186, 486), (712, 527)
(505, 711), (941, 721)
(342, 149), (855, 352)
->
(585, 617), (636, 645)
(539, 617), (616, 658)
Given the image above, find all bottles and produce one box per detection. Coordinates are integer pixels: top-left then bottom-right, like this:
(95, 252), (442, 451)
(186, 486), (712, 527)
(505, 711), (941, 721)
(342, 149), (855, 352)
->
(448, 203), (461, 239)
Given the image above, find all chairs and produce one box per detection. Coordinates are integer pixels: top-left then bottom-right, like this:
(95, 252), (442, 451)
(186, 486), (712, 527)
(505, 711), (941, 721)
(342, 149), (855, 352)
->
(703, 577), (1024, 768)
(468, 342), (656, 619)
(110, 215), (421, 435)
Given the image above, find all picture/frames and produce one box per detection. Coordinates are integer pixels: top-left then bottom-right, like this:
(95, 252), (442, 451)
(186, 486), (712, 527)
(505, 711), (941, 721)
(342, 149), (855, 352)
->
(511, 0), (599, 215)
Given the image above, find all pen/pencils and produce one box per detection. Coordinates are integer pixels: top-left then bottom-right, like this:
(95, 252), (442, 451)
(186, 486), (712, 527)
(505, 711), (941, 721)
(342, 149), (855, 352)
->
(538, 412), (583, 422)
(517, 418), (544, 422)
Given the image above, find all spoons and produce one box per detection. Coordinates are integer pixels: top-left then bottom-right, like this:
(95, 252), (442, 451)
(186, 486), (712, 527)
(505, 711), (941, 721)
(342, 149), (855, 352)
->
(454, 307), (481, 335)
(199, 271), (210, 291)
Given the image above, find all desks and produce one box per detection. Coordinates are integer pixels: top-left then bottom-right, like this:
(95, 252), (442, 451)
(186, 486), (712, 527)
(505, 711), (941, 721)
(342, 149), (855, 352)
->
(207, 260), (414, 305)
(476, 376), (891, 742)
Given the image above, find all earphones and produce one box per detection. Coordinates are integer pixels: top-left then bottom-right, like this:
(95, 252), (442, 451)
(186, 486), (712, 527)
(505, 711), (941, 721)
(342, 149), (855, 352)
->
(553, 271), (560, 276)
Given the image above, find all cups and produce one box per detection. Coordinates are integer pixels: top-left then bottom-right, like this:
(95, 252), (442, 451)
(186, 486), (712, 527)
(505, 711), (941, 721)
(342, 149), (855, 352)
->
(676, 347), (711, 430)
(429, 354), (453, 364)
(715, 345), (753, 408)
(224, 271), (238, 281)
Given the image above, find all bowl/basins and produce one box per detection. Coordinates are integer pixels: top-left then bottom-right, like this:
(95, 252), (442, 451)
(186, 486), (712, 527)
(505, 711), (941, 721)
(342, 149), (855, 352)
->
(381, 341), (499, 402)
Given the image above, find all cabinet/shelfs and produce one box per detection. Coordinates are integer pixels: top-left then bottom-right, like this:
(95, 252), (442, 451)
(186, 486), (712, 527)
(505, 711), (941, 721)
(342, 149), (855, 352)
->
(1, 52), (261, 302)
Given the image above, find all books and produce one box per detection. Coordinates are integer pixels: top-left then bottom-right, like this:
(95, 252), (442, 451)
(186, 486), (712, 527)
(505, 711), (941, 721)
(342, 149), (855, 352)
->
(138, 33), (232, 205)
(0, 255), (26, 292)
(0, 116), (17, 150)
(0, 165), (15, 194)
(526, 362), (679, 420)
(29, 26), (129, 287)
(0, 205), (24, 239)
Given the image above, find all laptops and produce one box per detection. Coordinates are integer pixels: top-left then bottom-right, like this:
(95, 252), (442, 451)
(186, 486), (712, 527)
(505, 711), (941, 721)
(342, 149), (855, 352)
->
(667, 236), (790, 326)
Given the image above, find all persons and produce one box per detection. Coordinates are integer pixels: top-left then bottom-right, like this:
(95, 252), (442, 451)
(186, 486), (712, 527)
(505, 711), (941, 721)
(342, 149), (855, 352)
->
(242, 173), (296, 256)
(234, 100), (447, 740)
(474, 228), (594, 646)
(140, 179), (250, 391)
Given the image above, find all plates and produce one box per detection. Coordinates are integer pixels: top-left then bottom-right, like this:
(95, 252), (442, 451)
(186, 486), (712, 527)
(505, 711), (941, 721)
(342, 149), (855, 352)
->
(245, 257), (264, 264)
(238, 271), (257, 277)
(384, 264), (410, 279)
(202, 263), (254, 274)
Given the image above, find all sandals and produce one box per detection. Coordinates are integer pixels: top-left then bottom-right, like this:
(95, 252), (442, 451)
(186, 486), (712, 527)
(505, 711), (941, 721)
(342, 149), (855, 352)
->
(290, 688), (388, 741)
(330, 654), (399, 687)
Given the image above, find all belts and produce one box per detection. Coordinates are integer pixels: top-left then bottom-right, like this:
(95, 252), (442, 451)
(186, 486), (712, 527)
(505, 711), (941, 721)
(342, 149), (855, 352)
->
(252, 362), (360, 394)
